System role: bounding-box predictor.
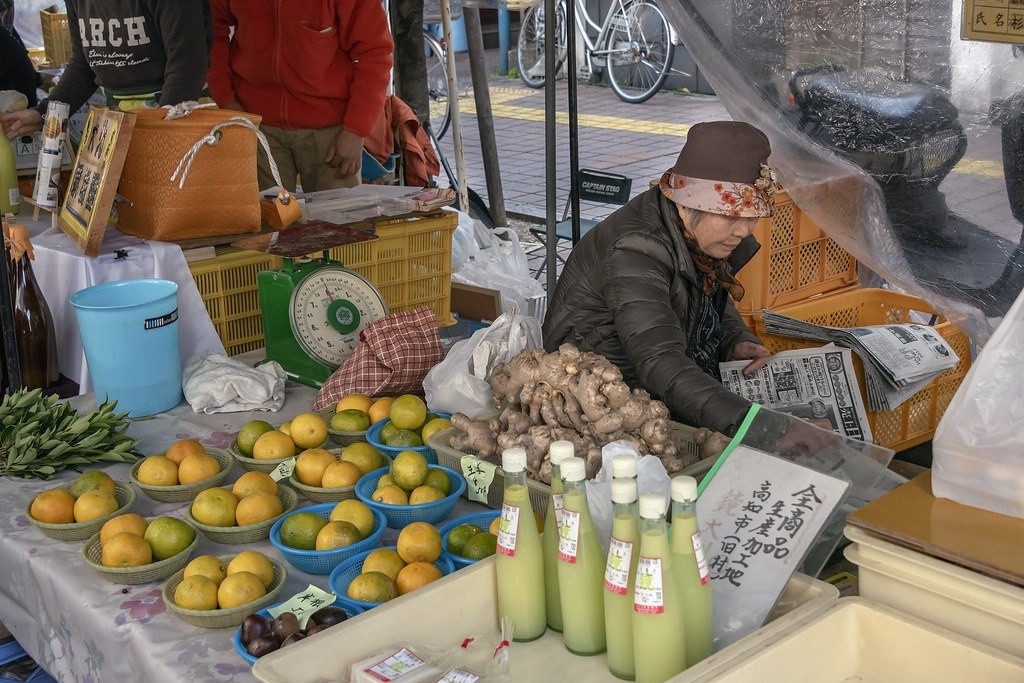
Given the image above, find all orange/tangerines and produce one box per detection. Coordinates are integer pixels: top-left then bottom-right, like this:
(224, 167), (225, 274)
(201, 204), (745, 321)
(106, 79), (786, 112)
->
(30, 395), (544, 609)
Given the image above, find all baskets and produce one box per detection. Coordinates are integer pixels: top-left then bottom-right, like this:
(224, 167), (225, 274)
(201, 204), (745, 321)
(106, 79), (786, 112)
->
(318, 398), (393, 446)
(269, 501), (388, 575)
(355, 463), (465, 529)
(287, 446), (392, 502)
(329, 546), (455, 610)
(365, 412), (449, 462)
(438, 511), (504, 569)
(26, 479), (135, 540)
(234, 599), (365, 665)
(228, 424), (329, 471)
(427, 420), (728, 517)
(187, 476), (298, 544)
(162, 552), (287, 629)
(128, 447), (233, 502)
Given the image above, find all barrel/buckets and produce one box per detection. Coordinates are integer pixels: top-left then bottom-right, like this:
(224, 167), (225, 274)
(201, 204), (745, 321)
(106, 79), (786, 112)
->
(69, 278), (182, 419)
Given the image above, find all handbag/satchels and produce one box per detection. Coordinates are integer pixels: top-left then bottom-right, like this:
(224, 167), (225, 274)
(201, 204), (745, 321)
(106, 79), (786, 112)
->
(115, 102), (300, 241)
(422, 312), (544, 417)
(82, 515), (200, 585)
(311, 306), (443, 411)
(441, 205), (547, 312)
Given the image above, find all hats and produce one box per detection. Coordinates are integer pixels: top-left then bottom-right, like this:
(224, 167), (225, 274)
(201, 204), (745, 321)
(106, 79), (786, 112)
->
(659, 121), (775, 218)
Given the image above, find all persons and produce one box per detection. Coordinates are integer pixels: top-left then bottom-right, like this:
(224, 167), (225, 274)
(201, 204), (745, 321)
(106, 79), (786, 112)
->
(540, 120), (838, 458)
(0, 0), (38, 110)
(207, 0), (394, 201)
(0, 0), (209, 139)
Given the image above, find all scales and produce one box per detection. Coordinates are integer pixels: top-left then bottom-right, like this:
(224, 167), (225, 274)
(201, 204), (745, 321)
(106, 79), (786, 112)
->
(231, 219), (388, 390)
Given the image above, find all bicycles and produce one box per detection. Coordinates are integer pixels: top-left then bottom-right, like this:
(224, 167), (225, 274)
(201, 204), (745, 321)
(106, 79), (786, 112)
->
(516, 0), (679, 104)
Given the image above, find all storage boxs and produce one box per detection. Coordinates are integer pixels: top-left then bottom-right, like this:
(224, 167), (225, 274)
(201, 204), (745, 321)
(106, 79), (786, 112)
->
(247, 524), (1024, 683)
(56, 105), (137, 257)
(426, 159), (973, 520)
(39, 10), (73, 68)
(187, 209), (459, 360)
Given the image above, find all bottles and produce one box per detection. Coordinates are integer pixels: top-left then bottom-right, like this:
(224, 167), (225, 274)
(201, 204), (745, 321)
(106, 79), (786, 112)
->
(495, 440), (714, 683)
(9, 225), (61, 394)
(1, 220), (15, 299)
(0, 119), (21, 216)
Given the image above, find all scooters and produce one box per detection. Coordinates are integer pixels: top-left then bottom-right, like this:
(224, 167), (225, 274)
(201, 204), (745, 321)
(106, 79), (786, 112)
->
(781, 60), (1024, 316)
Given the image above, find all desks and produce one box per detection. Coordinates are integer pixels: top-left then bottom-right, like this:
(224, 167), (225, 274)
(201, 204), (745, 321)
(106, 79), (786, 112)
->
(0, 335), (496, 683)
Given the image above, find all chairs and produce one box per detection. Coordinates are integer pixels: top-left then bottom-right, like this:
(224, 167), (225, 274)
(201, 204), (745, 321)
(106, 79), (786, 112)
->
(529, 168), (633, 280)
